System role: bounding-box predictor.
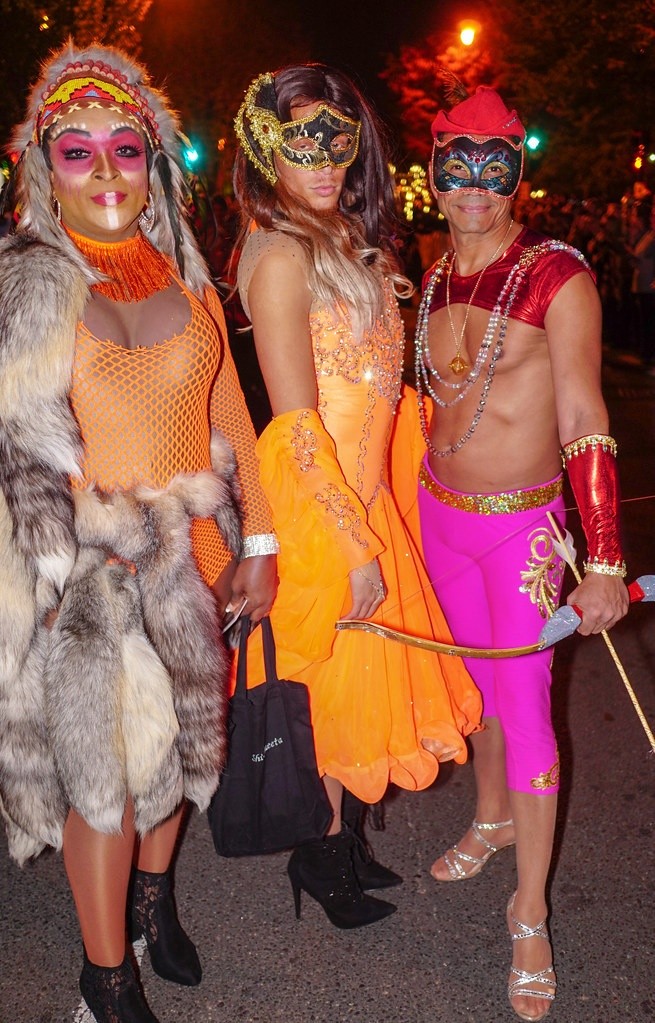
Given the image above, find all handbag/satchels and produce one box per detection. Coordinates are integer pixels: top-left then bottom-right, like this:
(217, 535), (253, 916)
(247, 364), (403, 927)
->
(205, 614), (333, 857)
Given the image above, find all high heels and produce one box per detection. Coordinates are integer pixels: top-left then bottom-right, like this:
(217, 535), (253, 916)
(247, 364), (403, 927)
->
(127, 862), (202, 986)
(286, 844), (398, 930)
(323, 821), (404, 888)
(78, 943), (158, 1023)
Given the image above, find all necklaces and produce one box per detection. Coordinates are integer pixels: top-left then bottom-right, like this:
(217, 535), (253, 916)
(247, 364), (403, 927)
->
(414, 219), (593, 459)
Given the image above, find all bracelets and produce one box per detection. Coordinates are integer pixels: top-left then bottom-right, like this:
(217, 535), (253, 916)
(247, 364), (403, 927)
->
(239, 533), (279, 559)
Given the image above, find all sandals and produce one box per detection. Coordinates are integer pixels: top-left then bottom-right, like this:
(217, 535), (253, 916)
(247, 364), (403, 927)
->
(505, 890), (557, 1022)
(429, 816), (517, 883)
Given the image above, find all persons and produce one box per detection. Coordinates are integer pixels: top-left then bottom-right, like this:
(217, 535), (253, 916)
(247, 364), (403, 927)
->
(237, 61), (485, 932)
(509, 182), (655, 298)
(417, 85), (630, 1021)
(0, 67), (280, 1023)
